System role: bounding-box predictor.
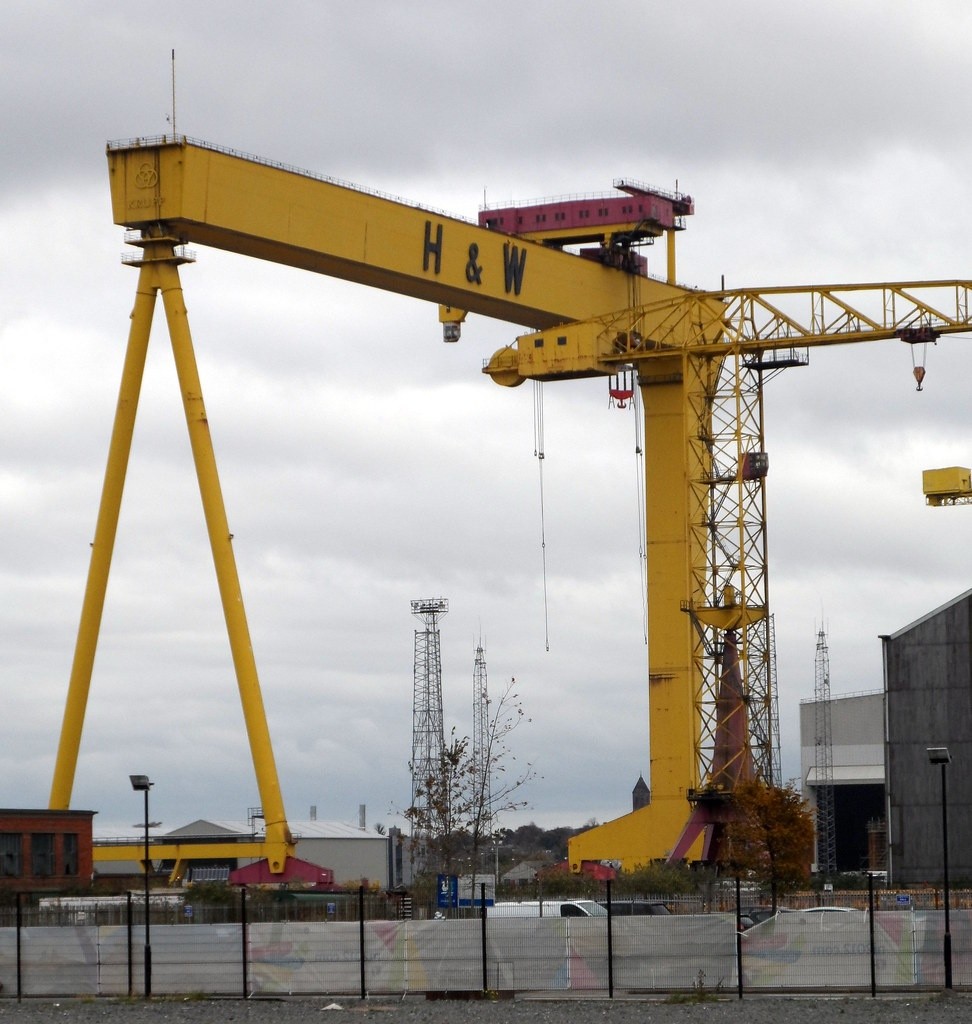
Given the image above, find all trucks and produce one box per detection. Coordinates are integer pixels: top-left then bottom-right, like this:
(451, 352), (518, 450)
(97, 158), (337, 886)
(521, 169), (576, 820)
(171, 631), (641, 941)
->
(487, 898), (607, 916)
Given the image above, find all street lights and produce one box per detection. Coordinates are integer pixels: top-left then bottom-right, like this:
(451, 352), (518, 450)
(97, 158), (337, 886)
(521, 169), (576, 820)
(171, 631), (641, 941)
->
(128, 774), (153, 999)
(926, 747), (952, 990)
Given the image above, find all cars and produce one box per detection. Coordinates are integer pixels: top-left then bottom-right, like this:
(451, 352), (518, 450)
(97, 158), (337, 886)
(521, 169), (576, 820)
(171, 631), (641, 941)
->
(725, 905), (861, 946)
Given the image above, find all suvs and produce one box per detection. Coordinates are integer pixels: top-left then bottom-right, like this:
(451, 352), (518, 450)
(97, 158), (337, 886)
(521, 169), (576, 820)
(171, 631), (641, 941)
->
(598, 899), (671, 916)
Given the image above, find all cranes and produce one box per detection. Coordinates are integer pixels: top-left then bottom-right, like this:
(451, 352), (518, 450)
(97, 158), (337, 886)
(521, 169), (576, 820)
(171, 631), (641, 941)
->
(47, 45), (971, 885)
